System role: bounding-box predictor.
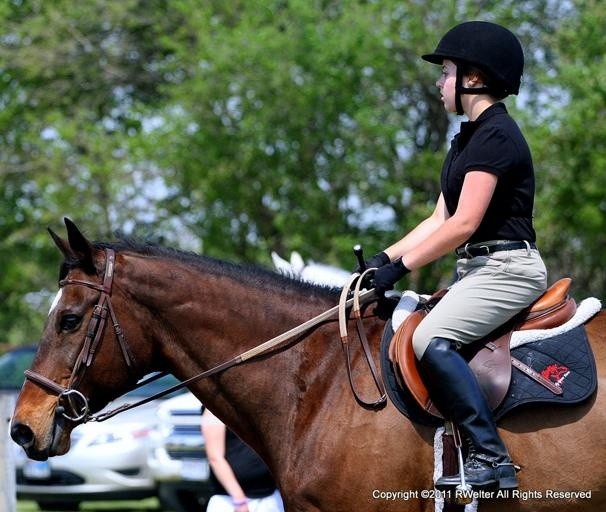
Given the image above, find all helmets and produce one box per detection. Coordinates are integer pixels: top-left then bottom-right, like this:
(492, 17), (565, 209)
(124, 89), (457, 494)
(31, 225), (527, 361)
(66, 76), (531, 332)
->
(421, 21), (524, 94)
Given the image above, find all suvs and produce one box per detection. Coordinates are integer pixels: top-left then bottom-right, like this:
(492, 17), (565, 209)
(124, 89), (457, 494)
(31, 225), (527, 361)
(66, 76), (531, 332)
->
(148, 387), (212, 512)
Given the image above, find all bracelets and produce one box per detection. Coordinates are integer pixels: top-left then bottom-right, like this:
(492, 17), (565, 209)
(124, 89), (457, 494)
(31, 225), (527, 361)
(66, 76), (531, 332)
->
(232, 500), (248, 506)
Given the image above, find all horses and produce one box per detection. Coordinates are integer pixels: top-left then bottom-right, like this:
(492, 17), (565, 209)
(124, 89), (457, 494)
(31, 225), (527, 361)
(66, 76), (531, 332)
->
(9, 216), (606, 512)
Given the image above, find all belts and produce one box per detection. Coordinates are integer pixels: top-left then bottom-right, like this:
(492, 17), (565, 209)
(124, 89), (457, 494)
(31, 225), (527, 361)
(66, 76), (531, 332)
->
(457, 241), (536, 261)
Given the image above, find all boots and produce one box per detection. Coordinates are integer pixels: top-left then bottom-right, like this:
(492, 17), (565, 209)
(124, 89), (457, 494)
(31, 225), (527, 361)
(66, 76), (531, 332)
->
(417, 336), (519, 489)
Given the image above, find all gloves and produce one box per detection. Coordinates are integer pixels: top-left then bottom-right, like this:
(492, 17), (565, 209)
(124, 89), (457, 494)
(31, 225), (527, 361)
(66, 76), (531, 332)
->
(349, 252), (411, 297)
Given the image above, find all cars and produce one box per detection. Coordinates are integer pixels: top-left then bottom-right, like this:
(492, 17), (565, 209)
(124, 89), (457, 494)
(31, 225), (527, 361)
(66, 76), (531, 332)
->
(15, 371), (188, 510)
(0, 344), (40, 510)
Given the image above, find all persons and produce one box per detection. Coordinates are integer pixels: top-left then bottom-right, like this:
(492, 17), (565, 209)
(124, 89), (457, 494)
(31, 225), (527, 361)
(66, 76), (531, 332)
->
(346, 19), (548, 489)
(199, 398), (288, 512)
